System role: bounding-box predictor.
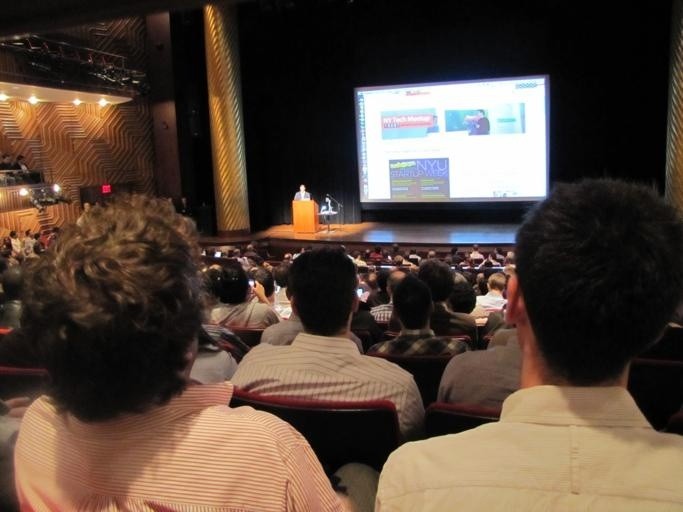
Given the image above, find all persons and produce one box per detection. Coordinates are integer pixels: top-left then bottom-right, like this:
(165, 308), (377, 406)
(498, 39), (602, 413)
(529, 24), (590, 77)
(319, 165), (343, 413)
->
(464, 109), (490, 137)
(376, 175), (682, 511)
(189, 242), (524, 443)
(320, 196), (335, 211)
(639, 311), (683, 362)
(2, 154), (34, 184)
(0, 389), (35, 512)
(294, 183), (311, 200)
(1, 203), (91, 328)
(13, 187), (380, 511)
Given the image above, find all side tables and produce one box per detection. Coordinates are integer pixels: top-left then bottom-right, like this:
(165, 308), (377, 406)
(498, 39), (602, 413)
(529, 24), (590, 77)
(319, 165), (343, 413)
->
(316, 212), (338, 234)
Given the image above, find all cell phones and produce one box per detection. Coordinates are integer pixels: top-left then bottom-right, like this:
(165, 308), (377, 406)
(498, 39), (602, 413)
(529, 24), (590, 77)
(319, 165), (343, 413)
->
(247, 278), (255, 294)
(357, 287), (364, 297)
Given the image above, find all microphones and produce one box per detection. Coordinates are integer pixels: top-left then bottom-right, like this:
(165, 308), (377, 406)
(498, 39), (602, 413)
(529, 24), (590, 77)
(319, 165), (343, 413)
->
(326, 194), (330, 197)
